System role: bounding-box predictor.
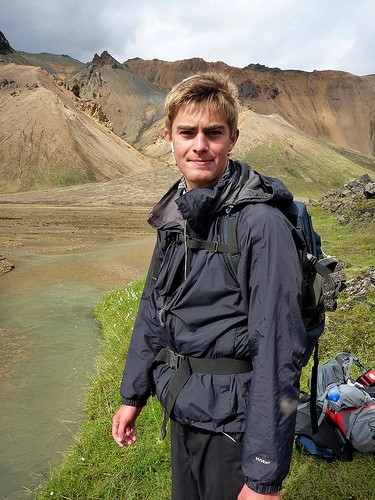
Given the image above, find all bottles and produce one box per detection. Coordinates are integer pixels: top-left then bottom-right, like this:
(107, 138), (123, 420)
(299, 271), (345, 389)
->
(328, 387), (340, 401)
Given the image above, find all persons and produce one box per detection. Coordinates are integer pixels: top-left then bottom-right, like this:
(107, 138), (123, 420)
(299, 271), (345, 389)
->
(112, 71), (308, 500)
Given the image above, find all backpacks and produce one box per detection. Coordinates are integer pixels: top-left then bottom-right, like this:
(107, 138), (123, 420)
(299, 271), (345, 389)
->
(148, 199), (339, 369)
(286, 351), (375, 463)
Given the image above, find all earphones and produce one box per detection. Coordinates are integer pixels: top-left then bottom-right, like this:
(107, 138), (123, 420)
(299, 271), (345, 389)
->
(170, 141), (174, 153)
